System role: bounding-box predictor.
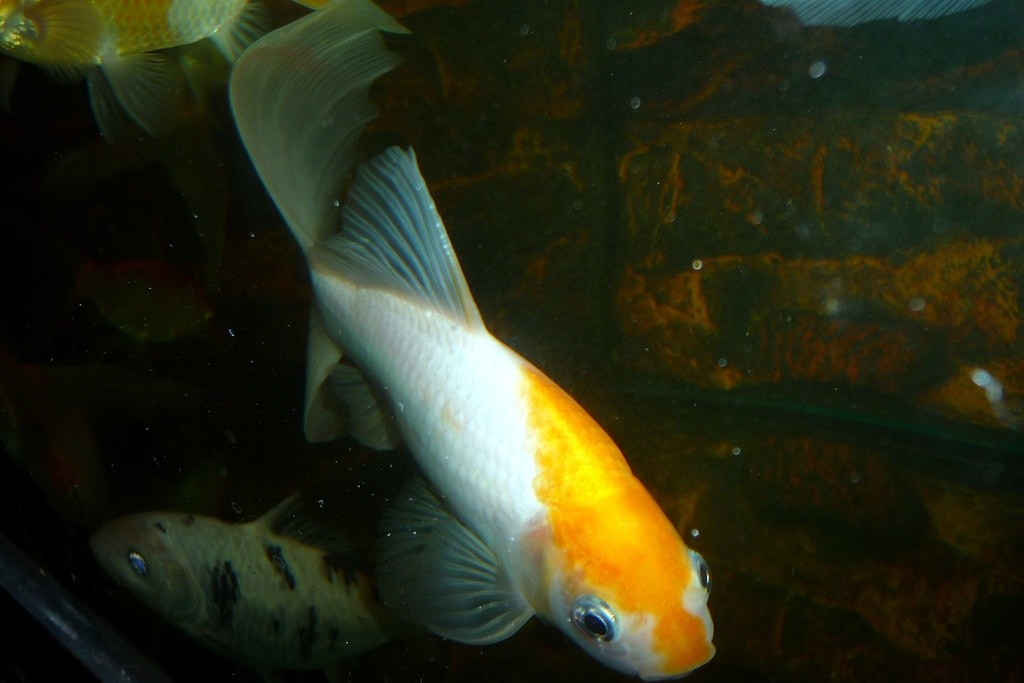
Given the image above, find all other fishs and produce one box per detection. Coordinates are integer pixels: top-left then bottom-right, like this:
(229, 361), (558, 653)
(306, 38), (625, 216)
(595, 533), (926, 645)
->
(87, 488), (418, 673)
(225, 3), (719, 681)
(0, 1), (414, 147)
